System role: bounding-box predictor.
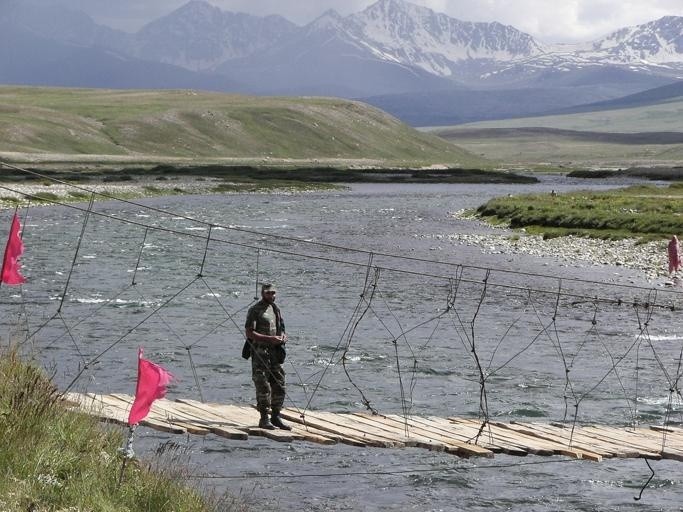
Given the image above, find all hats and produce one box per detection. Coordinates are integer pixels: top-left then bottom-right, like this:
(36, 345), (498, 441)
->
(262, 284), (276, 294)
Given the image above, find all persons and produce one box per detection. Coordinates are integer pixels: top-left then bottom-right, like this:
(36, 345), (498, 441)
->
(244, 283), (293, 431)
(668, 235), (679, 272)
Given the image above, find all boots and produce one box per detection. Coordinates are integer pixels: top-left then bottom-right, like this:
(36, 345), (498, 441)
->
(259, 413), (291, 431)
